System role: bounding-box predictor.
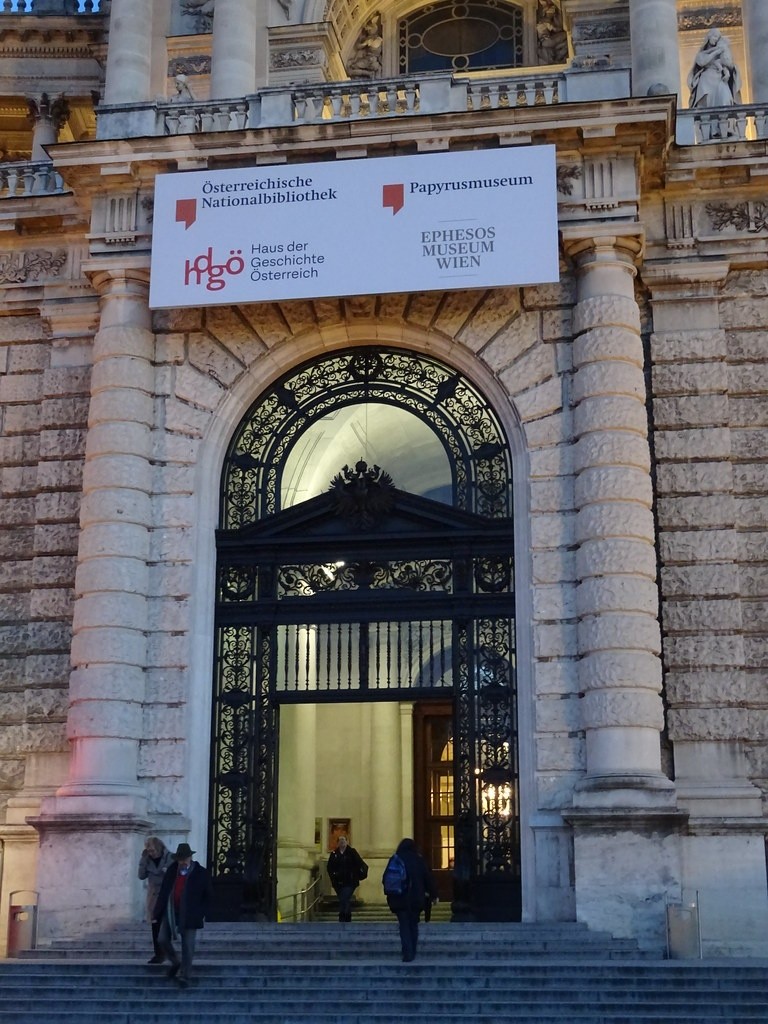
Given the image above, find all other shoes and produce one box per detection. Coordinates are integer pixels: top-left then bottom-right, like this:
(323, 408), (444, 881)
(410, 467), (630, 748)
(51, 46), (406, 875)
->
(171, 962), (180, 971)
(176, 977), (186, 984)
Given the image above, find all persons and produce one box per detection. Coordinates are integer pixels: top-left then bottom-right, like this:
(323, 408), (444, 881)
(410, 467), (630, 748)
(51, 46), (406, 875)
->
(384, 839), (437, 962)
(350, 23), (382, 79)
(153, 843), (211, 982)
(686, 28), (741, 138)
(537, 0), (567, 65)
(171, 75), (193, 102)
(327, 836), (363, 922)
(137, 835), (175, 963)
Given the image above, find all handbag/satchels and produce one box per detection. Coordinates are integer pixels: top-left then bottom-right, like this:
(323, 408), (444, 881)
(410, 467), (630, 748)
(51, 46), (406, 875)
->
(359, 861), (368, 880)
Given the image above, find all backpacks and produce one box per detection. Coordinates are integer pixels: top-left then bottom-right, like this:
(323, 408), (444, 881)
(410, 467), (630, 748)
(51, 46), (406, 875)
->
(384, 854), (407, 896)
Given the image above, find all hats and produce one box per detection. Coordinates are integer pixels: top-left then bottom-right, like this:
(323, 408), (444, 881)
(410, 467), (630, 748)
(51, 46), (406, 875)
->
(170, 843), (196, 861)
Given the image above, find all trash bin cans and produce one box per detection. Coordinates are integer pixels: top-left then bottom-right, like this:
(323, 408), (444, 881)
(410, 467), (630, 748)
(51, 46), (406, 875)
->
(6, 889), (39, 958)
(665, 885), (702, 958)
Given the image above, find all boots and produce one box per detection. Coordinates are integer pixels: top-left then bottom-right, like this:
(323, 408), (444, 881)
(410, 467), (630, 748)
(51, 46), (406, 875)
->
(147, 923), (165, 964)
